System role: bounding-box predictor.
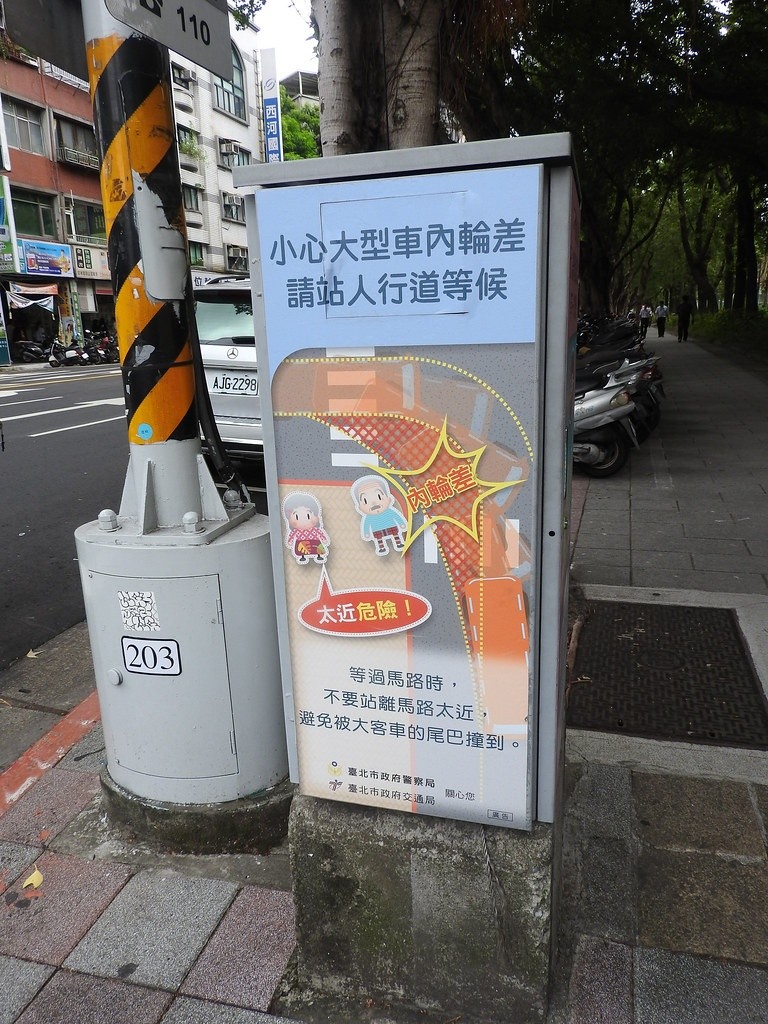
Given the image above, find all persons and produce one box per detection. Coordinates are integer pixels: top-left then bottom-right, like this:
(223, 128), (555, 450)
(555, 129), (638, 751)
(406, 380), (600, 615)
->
(59, 248), (70, 274)
(639, 299), (670, 339)
(677, 295), (694, 343)
(66, 324), (73, 346)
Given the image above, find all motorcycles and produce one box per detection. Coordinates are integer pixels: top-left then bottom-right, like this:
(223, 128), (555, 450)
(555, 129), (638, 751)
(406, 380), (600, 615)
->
(15, 327), (120, 368)
(570, 308), (670, 471)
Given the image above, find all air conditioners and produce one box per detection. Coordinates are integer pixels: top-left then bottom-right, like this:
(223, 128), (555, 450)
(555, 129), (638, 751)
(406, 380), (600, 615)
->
(180, 70), (198, 82)
(231, 247), (246, 258)
(226, 196), (241, 207)
(221, 143), (240, 156)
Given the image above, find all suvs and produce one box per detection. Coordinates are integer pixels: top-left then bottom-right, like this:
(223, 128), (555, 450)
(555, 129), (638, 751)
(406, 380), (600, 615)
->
(191, 274), (265, 461)
